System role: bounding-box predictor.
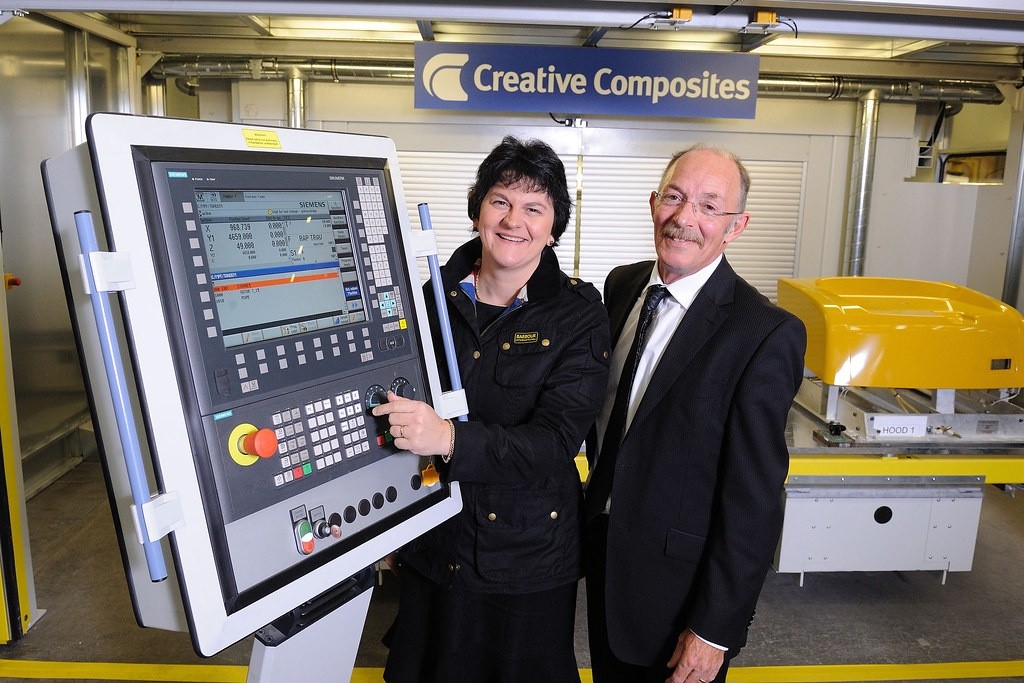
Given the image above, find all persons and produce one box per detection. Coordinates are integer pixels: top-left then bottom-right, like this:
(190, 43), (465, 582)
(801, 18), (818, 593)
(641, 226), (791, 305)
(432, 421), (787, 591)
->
(371, 136), (613, 683)
(581, 143), (807, 683)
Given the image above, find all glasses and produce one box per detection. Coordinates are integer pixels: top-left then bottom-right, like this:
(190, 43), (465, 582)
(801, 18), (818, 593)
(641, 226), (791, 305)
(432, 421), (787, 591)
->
(653, 187), (741, 218)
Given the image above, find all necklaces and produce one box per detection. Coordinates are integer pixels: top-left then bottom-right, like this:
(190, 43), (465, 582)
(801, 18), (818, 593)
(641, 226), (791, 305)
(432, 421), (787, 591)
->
(474, 267), (484, 301)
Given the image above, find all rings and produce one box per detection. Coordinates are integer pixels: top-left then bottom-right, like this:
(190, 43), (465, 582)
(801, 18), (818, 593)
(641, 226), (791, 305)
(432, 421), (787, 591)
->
(699, 678), (707, 683)
(399, 425), (406, 439)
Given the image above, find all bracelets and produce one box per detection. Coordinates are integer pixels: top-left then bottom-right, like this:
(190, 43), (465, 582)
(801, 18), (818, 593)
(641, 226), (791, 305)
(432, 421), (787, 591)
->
(442, 417), (457, 464)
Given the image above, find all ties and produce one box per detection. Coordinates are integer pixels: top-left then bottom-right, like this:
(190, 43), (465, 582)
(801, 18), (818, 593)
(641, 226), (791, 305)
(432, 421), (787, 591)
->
(582, 284), (675, 519)
